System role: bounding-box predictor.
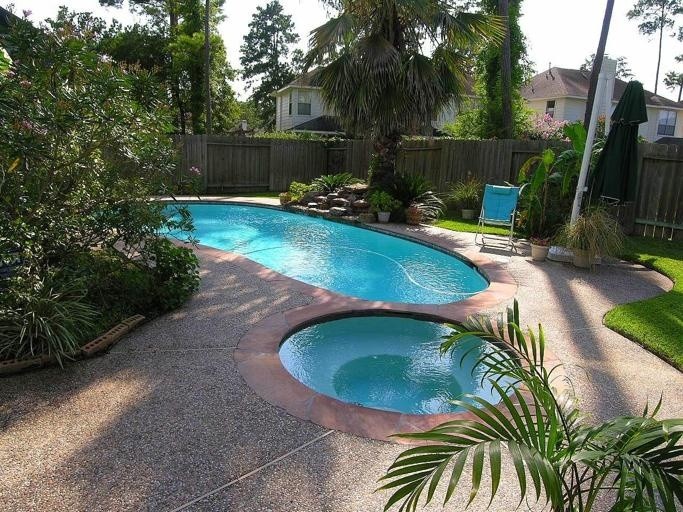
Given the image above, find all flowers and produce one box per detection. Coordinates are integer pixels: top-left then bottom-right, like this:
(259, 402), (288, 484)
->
(529, 234), (552, 246)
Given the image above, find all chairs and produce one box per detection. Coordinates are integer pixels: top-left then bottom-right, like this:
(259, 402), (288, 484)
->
(475, 183), (521, 253)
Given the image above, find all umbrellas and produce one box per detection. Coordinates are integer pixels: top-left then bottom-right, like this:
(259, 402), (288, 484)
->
(590, 80), (647, 234)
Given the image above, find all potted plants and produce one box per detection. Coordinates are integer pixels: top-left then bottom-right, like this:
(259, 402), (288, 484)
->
(367, 192), (403, 223)
(554, 208), (628, 270)
(441, 178), (484, 220)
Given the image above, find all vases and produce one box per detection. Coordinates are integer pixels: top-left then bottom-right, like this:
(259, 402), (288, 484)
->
(531, 244), (550, 261)
